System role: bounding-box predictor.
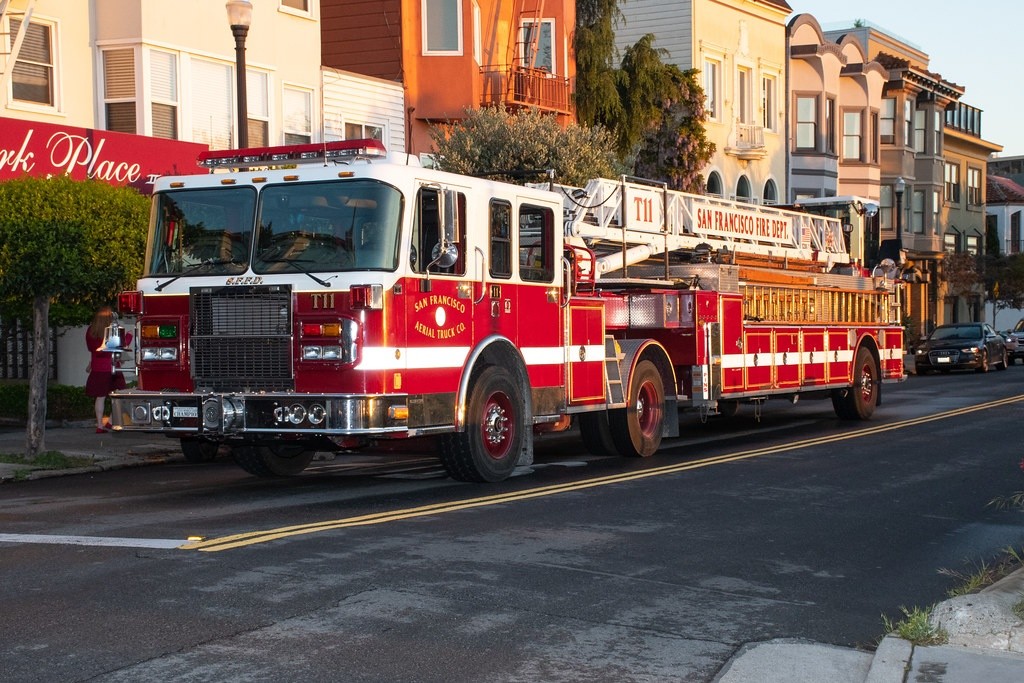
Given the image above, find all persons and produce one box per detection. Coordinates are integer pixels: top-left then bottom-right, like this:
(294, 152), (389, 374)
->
(86, 305), (134, 432)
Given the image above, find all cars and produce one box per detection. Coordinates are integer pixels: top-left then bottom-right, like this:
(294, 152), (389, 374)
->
(1005, 317), (1024, 367)
(911, 323), (1008, 376)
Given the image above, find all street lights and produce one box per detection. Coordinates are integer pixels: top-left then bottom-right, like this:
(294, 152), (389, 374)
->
(225, 1), (254, 171)
(893, 174), (905, 239)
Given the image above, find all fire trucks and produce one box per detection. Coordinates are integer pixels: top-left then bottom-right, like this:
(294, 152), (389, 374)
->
(96, 139), (909, 485)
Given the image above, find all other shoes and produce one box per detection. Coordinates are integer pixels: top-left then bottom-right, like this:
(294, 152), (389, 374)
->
(105, 423), (112, 429)
(96, 428), (108, 433)
